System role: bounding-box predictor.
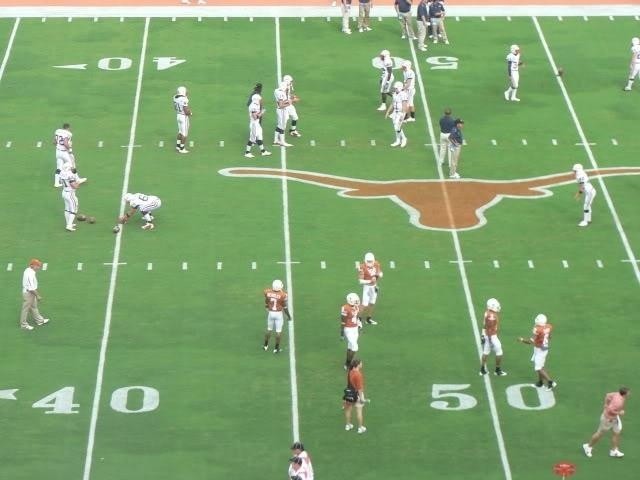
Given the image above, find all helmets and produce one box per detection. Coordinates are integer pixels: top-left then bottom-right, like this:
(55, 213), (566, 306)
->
(487, 298), (501, 313)
(511, 45), (519, 54)
(124, 193), (133, 202)
(632, 38), (640, 46)
(251, 75), (293, 104)
(346, 293), (360, 306)
(381, 50), (412, 92)
(364, 253), (375, 267)
(177, 86), (186, 96)
(535, 314), (547, 325)
(573, 164), (583, 172)
(272, 280), (283, 291)
(62, 162), (73, 173)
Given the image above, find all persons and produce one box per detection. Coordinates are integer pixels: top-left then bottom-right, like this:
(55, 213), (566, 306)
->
(292, 441), (314, 479)
(262, 279), (292, 353)
(289, 456), (312, 480)
(478, 297), (510, 375)
(173, 86), (192, 153)
(117, 193), (161, 231)
(245, 75), (301, 158)
(19, 257), (50, 331)
(378, 50), (416, 148)
(621, 37), (640, 91)
(501, 44), (524, 102)
(341, 0), (373, 35)
(340, 292), (363, 368)
(394, 0), (449, 52)
(582, 386), (632, 459)
(339, 359), (369, 433)
(571, 163), (595, 227)
(357, 252), (384, 325)
(54, 124), (87, 231)
(518, 314), (557, 390)
(438, 109), (467, 178)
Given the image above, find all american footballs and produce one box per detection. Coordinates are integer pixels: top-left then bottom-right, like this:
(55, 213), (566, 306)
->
(558, 67), (566, 79)
(88, 216), (97, 226)
(116, 214), (128, 226)
(77, 214), (86, 222)
(112, 224), (122, 234)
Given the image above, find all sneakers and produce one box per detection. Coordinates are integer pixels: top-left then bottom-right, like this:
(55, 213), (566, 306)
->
(20, 318), (49, 330)
(583, 444), (592, 458)
(54, 178), (86, 188)
(66, 224), (76, 231)
(531, 382), (544, 390)
(625, 86), (632, 91)
(609, 449), (624, 457)
(439, 160), (460, 179)
(546, 382), (557, 391)
(343, 27), (372, 33)
(376, 102), (416, 122)
(504, 93), (520, 101)
(263, 345), (283, 353)
(402, 33), (449, 51)
(478, 370), (490, 376)
(358, 426), (367, 434)
(579, 221), (588, 226)
(142, 216), (154, 228)
(345, 424), (353, 430)
(391, 139), (406, 147)
(494, 370), (507, 376)
(176, 146), (189, 153)
(366, 318), (377, 324)
(245, 130), (301, 158)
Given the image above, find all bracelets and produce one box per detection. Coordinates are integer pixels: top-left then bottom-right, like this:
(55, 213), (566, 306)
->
(521, 336), (524, 344)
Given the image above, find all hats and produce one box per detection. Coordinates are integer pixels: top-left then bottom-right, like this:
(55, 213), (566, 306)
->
(455, 118), (464, 124)
(290, 443), (304, 449)
(30, 259), (42, 266)
(290, 456), (302, 464)
(291, 475), (302, 480)
